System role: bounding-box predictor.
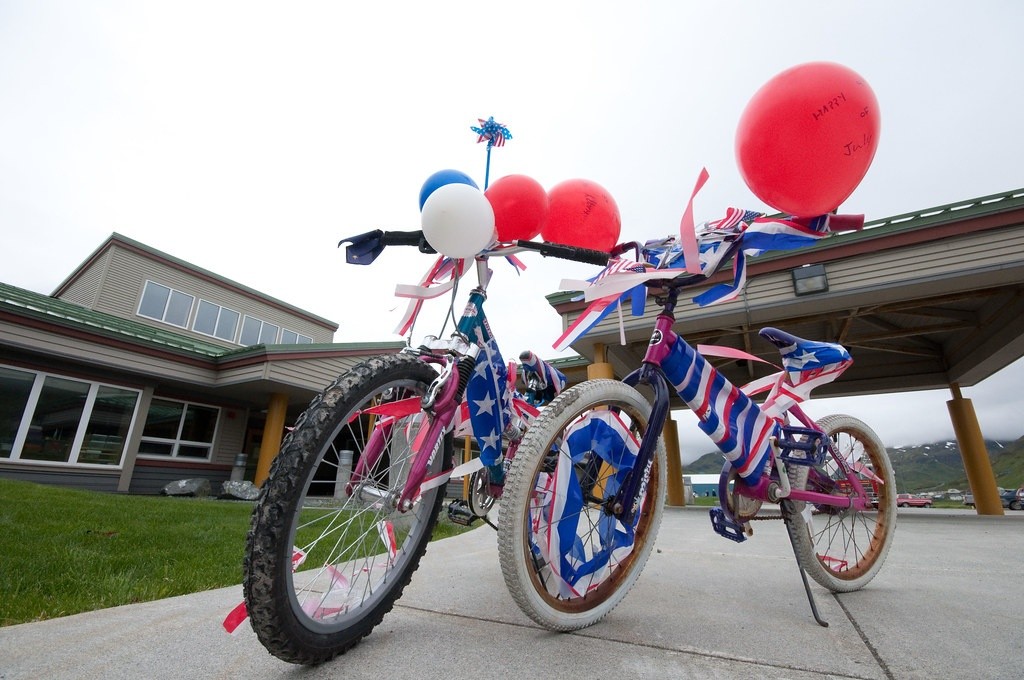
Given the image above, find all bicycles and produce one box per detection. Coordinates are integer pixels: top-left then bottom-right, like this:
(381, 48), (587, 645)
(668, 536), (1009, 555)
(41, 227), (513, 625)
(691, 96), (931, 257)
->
(496, 208), (899, 634)
(244, 225), (551, 668)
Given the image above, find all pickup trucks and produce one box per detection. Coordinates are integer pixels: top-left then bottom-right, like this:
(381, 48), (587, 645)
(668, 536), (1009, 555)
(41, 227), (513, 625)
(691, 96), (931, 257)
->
(961, 488), (1024, 511)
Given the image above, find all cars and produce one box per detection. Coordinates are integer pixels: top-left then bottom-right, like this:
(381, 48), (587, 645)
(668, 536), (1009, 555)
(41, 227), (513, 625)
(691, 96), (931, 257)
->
(897, 493), (933, 509)
